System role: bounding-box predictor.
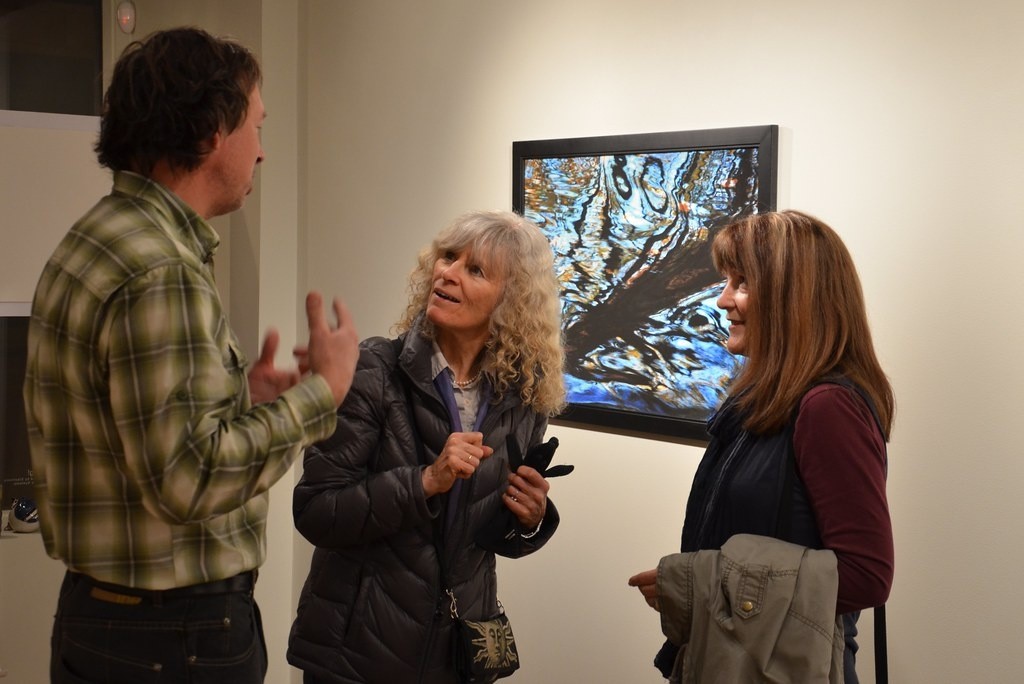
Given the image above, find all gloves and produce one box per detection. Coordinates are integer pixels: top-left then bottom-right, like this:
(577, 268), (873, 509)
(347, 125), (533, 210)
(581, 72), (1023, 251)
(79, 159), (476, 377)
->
(494, 431), (567, 546)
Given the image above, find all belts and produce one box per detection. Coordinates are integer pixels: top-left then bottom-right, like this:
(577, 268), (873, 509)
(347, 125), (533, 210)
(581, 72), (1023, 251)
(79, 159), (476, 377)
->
(66, 570), (253, 598)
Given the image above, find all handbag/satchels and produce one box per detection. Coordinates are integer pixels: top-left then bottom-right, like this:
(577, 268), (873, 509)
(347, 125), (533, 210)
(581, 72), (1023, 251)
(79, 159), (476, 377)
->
(447, 611), (521, 684)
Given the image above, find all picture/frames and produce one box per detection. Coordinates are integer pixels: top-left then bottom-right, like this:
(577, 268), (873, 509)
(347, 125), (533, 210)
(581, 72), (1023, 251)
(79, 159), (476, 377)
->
(512, 123), (781, 446)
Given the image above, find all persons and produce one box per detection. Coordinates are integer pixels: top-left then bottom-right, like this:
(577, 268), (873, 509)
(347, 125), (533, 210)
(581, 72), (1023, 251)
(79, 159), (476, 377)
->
(21, 24), (360, 684)
(287, 207), (574, 684)
(628, 209), (897, 684)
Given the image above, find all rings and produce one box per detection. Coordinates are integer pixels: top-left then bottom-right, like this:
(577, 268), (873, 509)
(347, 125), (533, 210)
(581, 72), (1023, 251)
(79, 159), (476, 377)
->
(516, 490), (519, 498)
(465, 455), (472, 463)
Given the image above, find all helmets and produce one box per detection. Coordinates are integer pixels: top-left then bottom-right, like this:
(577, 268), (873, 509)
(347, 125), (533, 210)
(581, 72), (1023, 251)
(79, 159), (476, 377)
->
(8, 498), (42, 535)
